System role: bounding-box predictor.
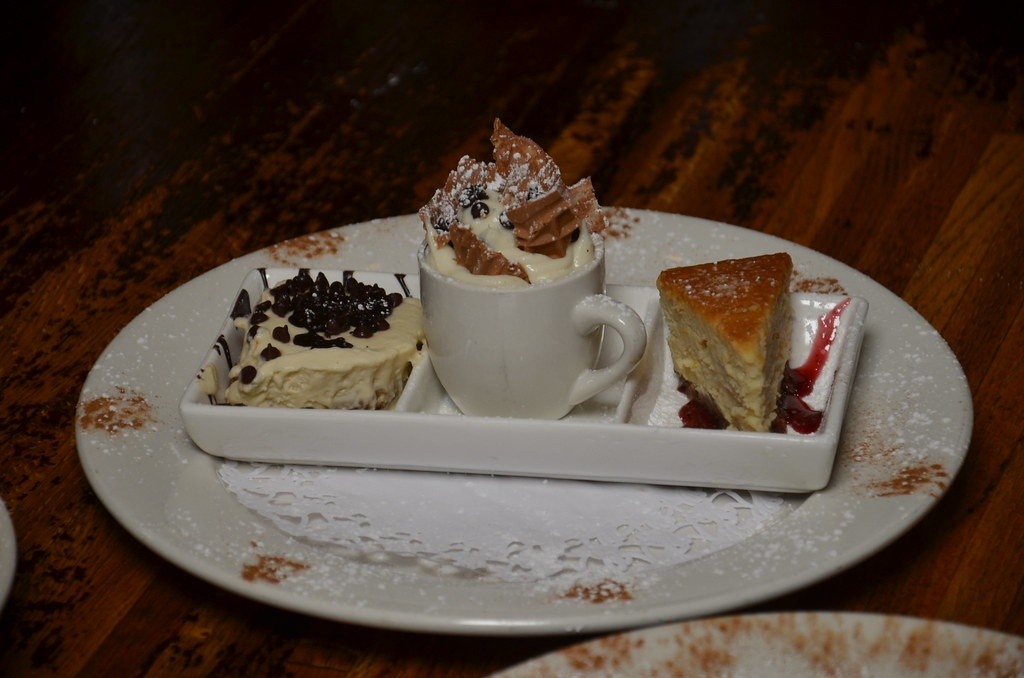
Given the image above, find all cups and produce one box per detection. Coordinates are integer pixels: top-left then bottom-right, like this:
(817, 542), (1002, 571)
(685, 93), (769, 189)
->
(417, 235), (649, 421)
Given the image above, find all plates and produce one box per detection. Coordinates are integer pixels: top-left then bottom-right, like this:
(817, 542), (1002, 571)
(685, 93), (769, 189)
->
(180, 266), (872, 494)
(71, 205), (973, 636)
(488, 613), (1024, 677)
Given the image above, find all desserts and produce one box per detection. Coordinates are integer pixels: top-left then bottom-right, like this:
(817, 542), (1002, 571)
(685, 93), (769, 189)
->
(219, 276), (434, 419)
(655, 244), (795, 436)
(405, 118), (607, 291)
